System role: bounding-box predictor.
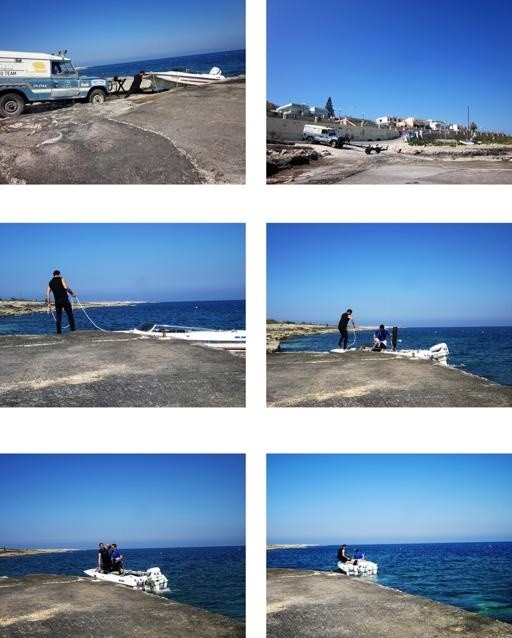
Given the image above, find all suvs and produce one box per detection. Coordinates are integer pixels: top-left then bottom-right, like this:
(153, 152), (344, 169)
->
(0, 48), (109, 119)
(303, 125), (346, 149)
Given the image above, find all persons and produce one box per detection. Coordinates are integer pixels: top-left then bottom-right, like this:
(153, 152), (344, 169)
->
(123, 70), (153, 98)
(372, 325), (393, 349)
(52, 62), (67, 76)
(46, 270), (77, 333)
(337, 309), (355, 350)
(337, 544), (351, 563)
(96, 542), (124, 575)
(351, 548), (365, 565)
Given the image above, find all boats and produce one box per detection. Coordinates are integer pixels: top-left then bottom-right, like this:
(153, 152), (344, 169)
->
(337, 558), (378, 577)
(83, 566), (169, 593)
(330, 342), (450, 367)
(113, 323), (246, 360)
(144, 66), (225, 91)
